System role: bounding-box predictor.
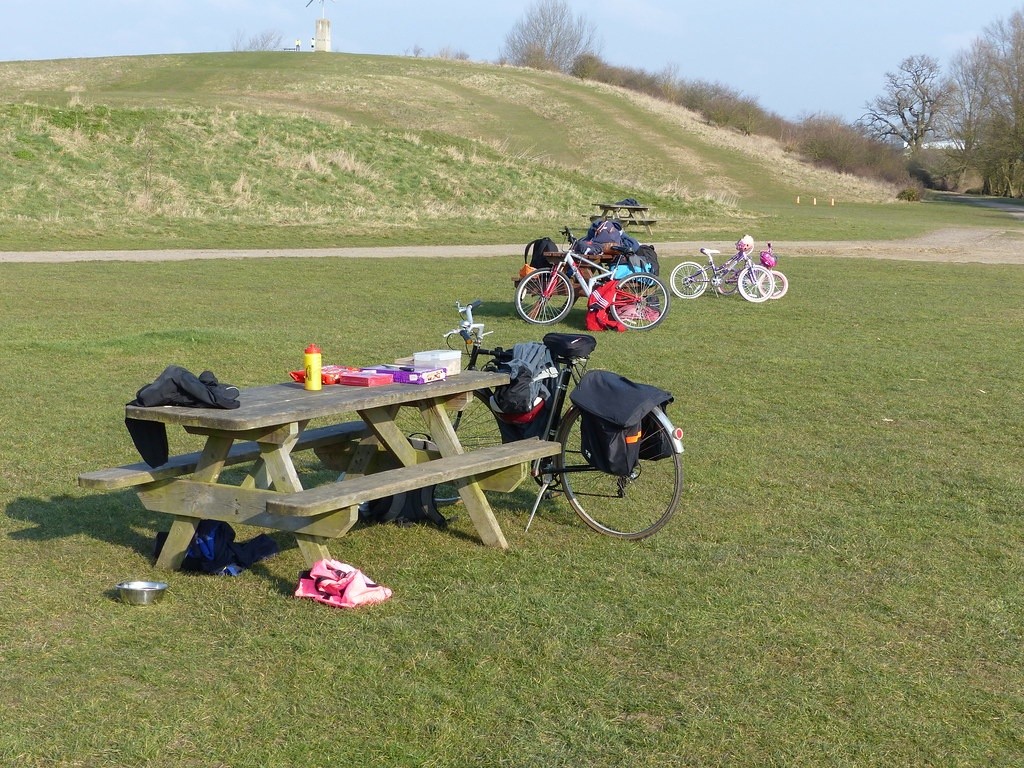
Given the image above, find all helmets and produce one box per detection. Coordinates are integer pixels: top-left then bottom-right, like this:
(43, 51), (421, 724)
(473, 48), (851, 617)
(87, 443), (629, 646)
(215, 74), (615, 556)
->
(736, 234), (754, 252)
(491, 396), (543, 423)
(760, 251), (777, 267)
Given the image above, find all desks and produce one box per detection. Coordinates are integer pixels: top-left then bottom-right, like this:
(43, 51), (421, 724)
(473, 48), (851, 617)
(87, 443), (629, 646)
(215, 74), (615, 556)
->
(124, 371), (509, 569)
(592, 203), (653, 236)
(543, 251), (612, 314)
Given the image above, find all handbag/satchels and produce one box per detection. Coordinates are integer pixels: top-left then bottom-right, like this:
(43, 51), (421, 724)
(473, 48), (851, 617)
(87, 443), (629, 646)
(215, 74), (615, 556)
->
(157, 520), (236, 573)
(569, 369), (674, 477)
(576, 221), (660, 286)
(296, 559), (392, 608)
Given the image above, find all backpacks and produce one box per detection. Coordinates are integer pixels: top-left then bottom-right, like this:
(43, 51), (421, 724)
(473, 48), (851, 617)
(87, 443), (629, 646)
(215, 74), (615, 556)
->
(524, 237), (559, 269)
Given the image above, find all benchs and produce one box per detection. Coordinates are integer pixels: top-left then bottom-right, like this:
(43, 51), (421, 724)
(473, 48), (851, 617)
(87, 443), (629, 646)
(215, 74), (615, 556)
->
(77, 421), (368, 490)
(511, 276), (641, 297)
(605, 217), (656, 226)
(264, 438), (562, 517)
(582, 214), (628, 218)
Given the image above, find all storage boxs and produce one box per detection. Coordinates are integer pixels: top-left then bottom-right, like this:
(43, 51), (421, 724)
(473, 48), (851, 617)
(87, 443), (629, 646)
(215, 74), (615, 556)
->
(413, 350), (462, 375)
(339, 372), (394, 387)
(360, 364), (447, 385)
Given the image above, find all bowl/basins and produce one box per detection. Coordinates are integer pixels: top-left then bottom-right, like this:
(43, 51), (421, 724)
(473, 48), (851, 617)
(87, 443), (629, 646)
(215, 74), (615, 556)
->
(115, 580), (168, 606)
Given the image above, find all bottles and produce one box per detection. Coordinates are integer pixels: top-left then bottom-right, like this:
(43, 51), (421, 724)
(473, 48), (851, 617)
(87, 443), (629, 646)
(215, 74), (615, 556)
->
(304, 343), (322, 391)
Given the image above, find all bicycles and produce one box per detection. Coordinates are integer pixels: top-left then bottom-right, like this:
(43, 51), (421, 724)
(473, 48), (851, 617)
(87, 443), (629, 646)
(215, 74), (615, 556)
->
(719, 241), (789, 300)
(512, 225), (673, 334)
(670, 233), (775, 303)
(391, 298), (685, 542)
